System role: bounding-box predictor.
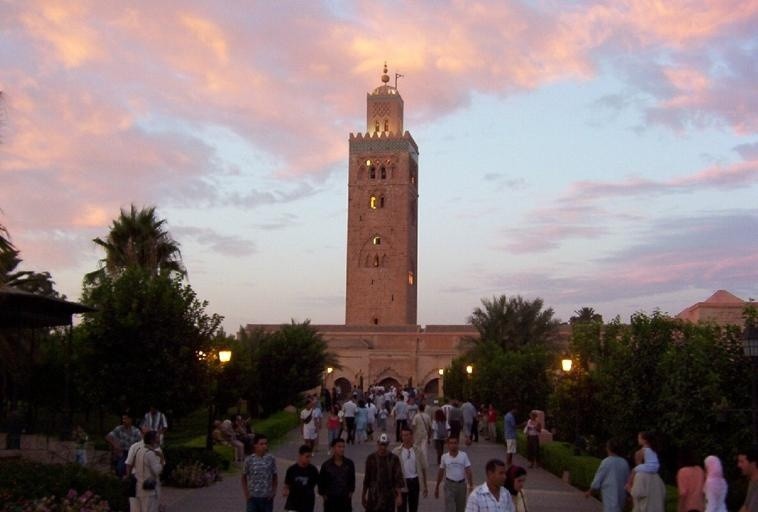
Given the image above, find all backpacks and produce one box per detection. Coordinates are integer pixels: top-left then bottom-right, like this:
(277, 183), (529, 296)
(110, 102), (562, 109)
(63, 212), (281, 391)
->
(437, 422), (448, 438)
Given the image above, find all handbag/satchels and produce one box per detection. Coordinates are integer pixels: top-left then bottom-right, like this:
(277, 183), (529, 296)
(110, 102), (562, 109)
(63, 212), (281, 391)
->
(523, 426), (528, 434)
(428, 432), (431, 444)
(304, 413), (312, 424)
(367, 427), (373, 435)
(143, 480), (156, 490)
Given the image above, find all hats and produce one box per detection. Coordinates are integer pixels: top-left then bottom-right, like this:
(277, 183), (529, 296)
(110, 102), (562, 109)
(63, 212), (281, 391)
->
(376, 433), (390, 445)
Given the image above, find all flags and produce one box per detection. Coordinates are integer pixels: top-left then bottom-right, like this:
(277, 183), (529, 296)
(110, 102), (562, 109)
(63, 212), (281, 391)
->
(397, 74), (405, 79)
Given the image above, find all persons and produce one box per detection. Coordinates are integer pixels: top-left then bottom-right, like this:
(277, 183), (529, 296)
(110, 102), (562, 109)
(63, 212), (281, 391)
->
(465, 459), (528, 511)
(105, 404), (169, 512)
(434, 436), (474, 511)
(736, 452), (758, 512)
(483, 404), (498, 442)
(624, 431), (661, 488)
(391, 427), (428, 511)
(361, 432), (405, 511)
(625, 452), (666, 512)
(586, 439), (630, 512)
(675, 443), (705, 512)
(284, 445), (319, 511)
(318, 438), (356, 511)
(241, 434), (279, 511)
(300, 382), (479, 466)
(213, 414), (255, 462)
(702, 454), (728, 512)
(524, 410), (542, 470)
(71, 426), (89, 463)
(505, 405), (518, 467)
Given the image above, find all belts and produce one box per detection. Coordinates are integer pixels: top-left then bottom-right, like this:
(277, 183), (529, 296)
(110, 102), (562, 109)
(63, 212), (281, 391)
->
(446, 478), (465, 483)
